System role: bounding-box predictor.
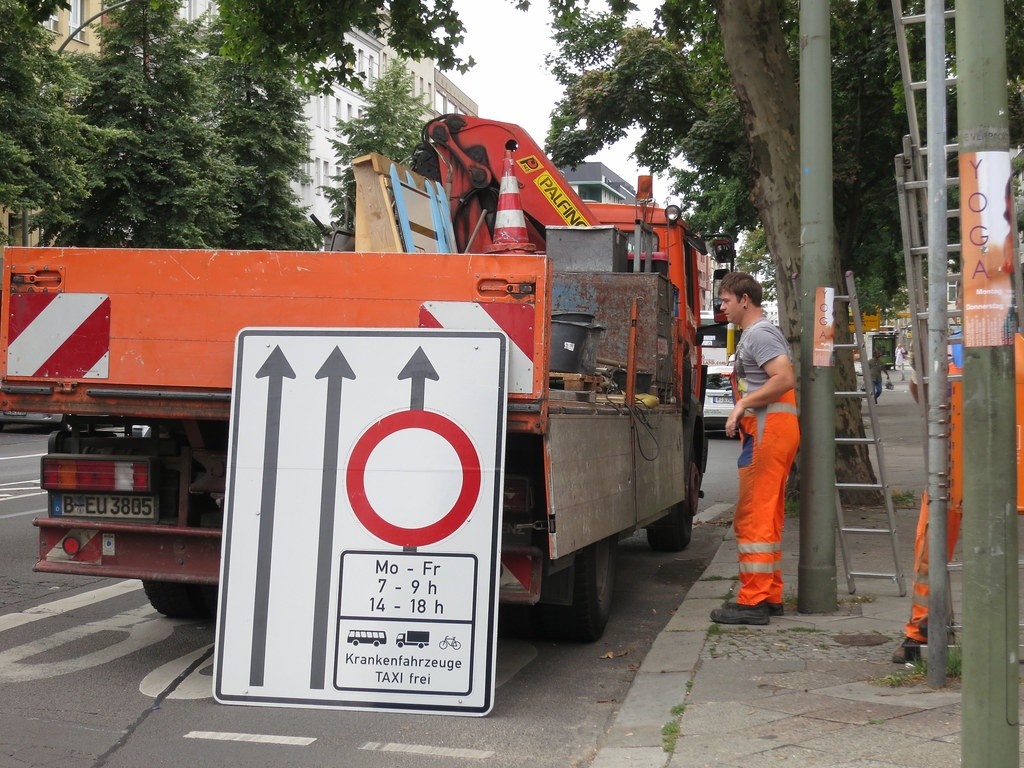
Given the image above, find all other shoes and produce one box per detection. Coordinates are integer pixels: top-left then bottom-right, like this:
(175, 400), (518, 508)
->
(722, 601), (784, 616)
(711, 604), (770, 625)
(892, 637), (928, 663)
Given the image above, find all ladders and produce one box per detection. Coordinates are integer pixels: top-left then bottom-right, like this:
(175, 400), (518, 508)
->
(888, 1), (964, 660)
(829, 269), (908, 598)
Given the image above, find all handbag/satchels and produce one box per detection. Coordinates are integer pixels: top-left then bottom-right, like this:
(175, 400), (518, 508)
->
(885, 378), (894, 390)
(875, 399), (878, 404)
(860, 383), (866, 392)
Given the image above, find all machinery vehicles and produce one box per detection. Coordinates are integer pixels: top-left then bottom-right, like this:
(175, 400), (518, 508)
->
(0, 113), (735, 644)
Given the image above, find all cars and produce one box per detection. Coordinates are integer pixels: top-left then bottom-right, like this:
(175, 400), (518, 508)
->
(701, 366), (742, 430)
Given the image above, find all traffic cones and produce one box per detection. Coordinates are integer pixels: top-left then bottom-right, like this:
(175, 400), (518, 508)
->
(483, 151), (542, 255)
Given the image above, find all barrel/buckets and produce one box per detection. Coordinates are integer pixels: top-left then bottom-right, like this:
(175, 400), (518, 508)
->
(618, 372), (653, 393)
(550, 313), (607, 375)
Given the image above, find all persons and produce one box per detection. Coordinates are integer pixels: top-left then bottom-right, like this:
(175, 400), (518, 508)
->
(869, 351), (889, 404)
(893, 280), (961, 664)
(709, 272), (801, 626)
(895, 344), (905, 369)
